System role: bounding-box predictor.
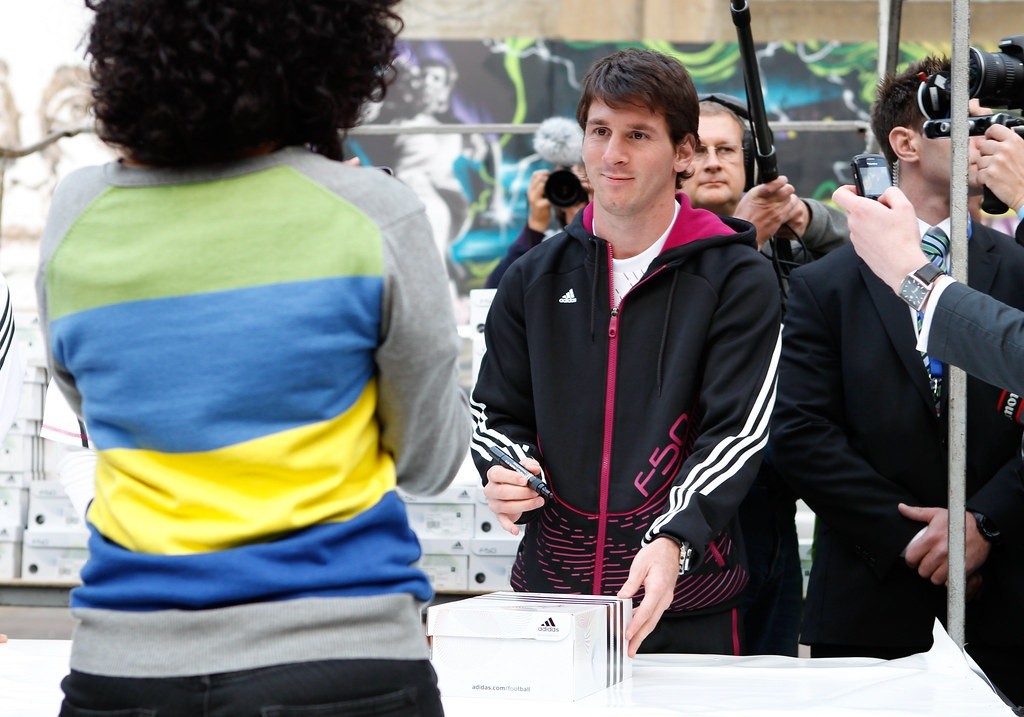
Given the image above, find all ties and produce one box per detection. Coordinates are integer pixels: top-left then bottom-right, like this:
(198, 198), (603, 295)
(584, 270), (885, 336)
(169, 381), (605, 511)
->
(919, 226), (950, 418)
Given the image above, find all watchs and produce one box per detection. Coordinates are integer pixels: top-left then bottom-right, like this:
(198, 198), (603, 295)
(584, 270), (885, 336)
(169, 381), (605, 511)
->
(971, 511), (1001, 540)
(651, 533), (693, 575)
(898, 262), (946, 313)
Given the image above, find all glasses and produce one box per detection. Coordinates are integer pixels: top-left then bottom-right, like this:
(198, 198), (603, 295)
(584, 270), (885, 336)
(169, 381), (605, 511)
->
(695, 144), (747, 161)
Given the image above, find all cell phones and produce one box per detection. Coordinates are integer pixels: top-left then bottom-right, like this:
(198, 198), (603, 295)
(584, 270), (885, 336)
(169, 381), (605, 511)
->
(852, 154), (893, 202)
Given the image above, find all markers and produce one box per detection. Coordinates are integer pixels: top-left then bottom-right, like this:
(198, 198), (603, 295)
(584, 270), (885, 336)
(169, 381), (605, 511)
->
(489, 446), (553, 498)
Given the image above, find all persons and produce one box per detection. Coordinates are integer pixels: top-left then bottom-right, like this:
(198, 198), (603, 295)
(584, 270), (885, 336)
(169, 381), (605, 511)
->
(36, 0), (474, 717)
(469, 47), (1024, 717)
(360, 55), (497, 295)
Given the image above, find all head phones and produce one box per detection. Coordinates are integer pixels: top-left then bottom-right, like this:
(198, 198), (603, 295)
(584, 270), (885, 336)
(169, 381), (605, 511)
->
(700, 92), (774, 192)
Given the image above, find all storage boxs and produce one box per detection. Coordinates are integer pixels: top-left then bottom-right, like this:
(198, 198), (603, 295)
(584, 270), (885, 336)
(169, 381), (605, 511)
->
(427, 592), (634, 699)
(0, 289), (526, 592)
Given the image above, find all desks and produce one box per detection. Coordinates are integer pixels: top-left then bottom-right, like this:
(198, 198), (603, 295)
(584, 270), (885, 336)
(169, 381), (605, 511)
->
(0, 615), (1024, 717)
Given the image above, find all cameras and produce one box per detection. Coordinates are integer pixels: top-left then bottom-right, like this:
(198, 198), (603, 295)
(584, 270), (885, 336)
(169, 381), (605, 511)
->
(544, 170), (589, 206)
(969, 35), (1024, 111)
